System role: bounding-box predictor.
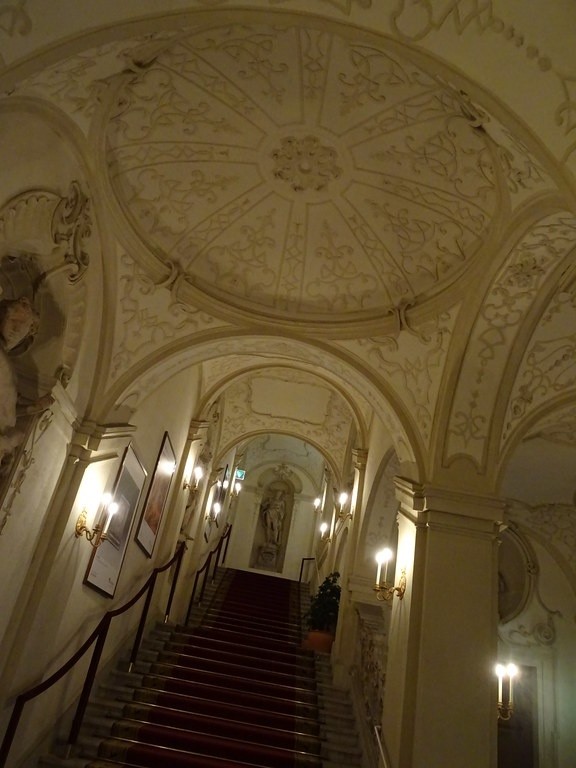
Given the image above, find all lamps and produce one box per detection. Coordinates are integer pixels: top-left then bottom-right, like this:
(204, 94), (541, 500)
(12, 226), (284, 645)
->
(75, 493), (119, 547)
(373, 548), (406, 601)
(320, 523), (332, 542)
(495, 661), (520, 721)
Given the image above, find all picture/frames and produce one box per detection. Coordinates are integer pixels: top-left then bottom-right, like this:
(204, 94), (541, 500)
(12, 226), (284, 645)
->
(82, 431), (176, 600)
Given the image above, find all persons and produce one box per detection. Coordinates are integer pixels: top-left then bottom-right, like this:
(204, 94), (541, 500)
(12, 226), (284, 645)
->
(0, 292), (56, 469)
(259, 489), (286, 545)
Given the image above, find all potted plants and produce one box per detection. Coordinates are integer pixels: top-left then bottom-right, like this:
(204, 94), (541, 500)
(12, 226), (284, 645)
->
(302, 572), (341, 653)
